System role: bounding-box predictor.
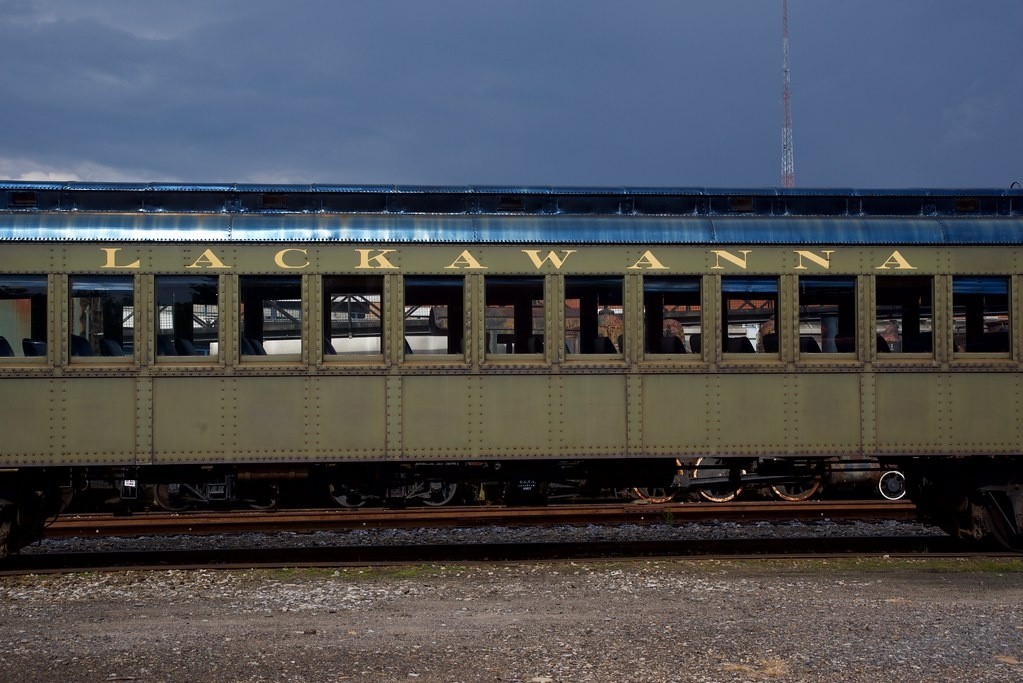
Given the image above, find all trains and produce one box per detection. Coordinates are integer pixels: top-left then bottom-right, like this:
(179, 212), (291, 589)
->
(1, 179), (1023, 558)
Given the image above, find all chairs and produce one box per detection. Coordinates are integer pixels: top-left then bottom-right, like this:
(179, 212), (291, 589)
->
(0, 328), (1014, 356)
